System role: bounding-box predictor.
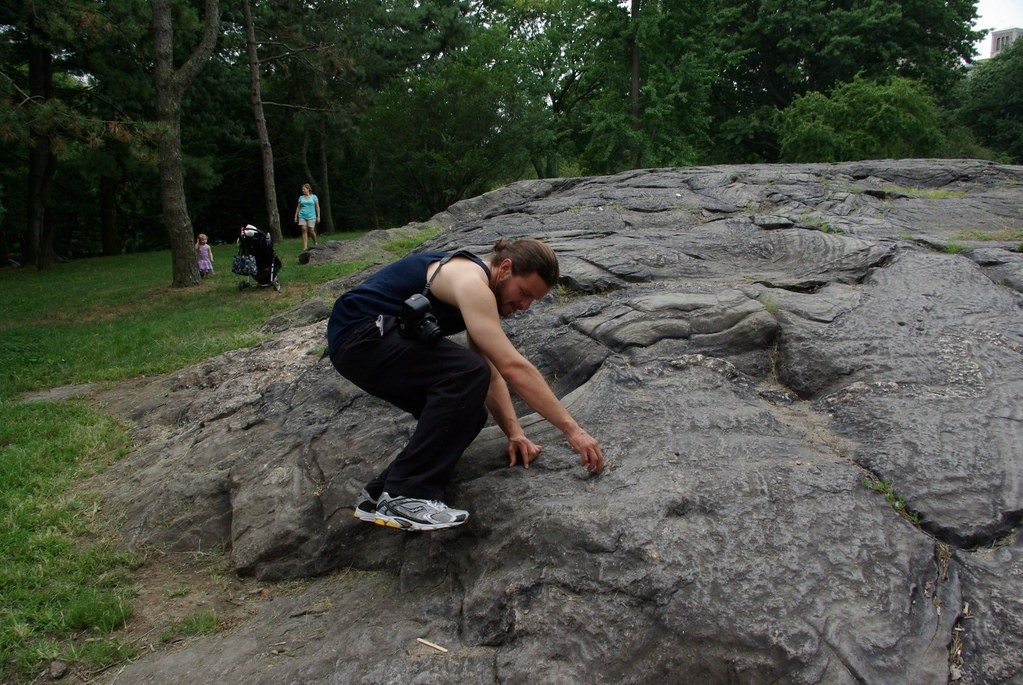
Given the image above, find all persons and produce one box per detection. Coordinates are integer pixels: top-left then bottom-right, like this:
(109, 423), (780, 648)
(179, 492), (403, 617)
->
(195, 234), (214, 279)
(294, 184), (321, 250)
(326, 236), (603, 531)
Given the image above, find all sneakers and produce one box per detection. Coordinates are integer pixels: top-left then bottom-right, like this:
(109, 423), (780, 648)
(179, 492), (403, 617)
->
(354, 486), (379, 522)
(374, 495), (470, 531)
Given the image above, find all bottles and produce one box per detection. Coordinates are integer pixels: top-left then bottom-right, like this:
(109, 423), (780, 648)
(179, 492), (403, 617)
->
(266, 233), (271, 247)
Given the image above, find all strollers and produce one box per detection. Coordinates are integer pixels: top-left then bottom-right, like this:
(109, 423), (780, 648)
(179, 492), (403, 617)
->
(231, 224), (283, 292)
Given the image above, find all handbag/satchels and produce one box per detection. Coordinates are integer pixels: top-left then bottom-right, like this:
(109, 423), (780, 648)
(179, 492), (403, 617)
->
(232, 256), (258, 275)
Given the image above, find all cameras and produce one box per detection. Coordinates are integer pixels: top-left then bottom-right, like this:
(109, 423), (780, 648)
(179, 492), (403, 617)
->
(397, 293), (442, 349)
